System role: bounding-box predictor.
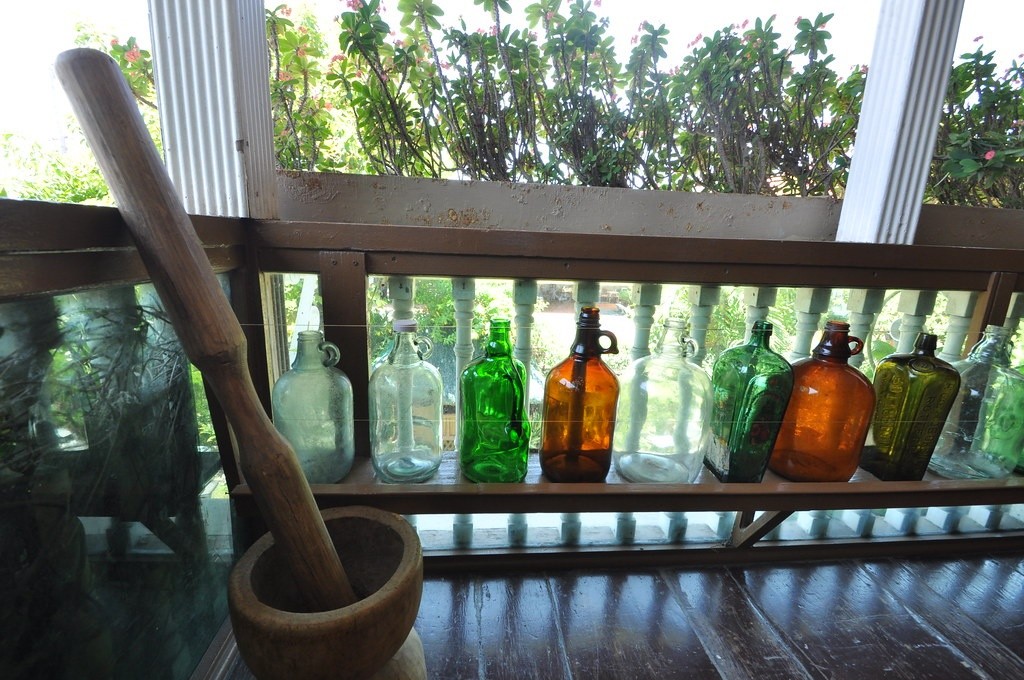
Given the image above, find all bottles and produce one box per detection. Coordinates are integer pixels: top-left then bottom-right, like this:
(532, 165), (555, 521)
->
(270, 331), (355, 485)
(370, 319), (443, 483)
(456, 318), (531, 484)
(539, 305), (1024, 484)
